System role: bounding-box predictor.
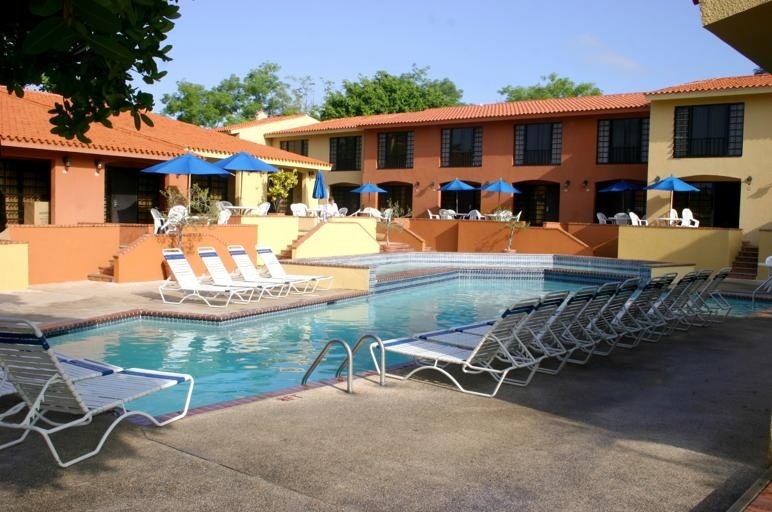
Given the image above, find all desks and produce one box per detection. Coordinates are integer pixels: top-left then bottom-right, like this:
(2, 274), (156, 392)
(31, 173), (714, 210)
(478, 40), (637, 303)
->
(752, 263), (772, 308)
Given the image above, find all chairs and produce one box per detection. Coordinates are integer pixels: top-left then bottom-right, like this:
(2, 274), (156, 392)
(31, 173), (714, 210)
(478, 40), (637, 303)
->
(597, 207), (700, 228)
(765, 256), (772, 293)
(0, 316), (194, 468)
(160, 245), (333, 308)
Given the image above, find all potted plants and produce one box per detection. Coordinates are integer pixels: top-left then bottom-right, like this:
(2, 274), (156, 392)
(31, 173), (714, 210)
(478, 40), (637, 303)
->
(488, 208), (531, 253)
(155, 183), (225, 281)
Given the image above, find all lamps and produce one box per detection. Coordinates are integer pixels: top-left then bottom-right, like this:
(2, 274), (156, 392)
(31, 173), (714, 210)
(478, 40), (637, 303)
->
(746, 176), (752, 185)
(583, 180), (590, 192)
(655, 176), (660, 183)
(94, 158), (102, 169)
(564, 180), (571, 192)
(62, 155), (72, 167)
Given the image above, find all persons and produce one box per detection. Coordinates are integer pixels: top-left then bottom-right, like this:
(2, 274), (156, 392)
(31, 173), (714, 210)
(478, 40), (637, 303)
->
(327, 197), (338, 215)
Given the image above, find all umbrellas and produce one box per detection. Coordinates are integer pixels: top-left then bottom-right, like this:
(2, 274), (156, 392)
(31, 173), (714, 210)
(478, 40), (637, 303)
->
(643, 175), (700, 226)
(213, 151), (280, 214)
(142, 154), (236, 216)
(600, 181), (640, 212)
(476, 179), (521, 214)
(436, 178), (475, 213)
(350, 180), (387, 217)
(313, 169), (328, 206)
